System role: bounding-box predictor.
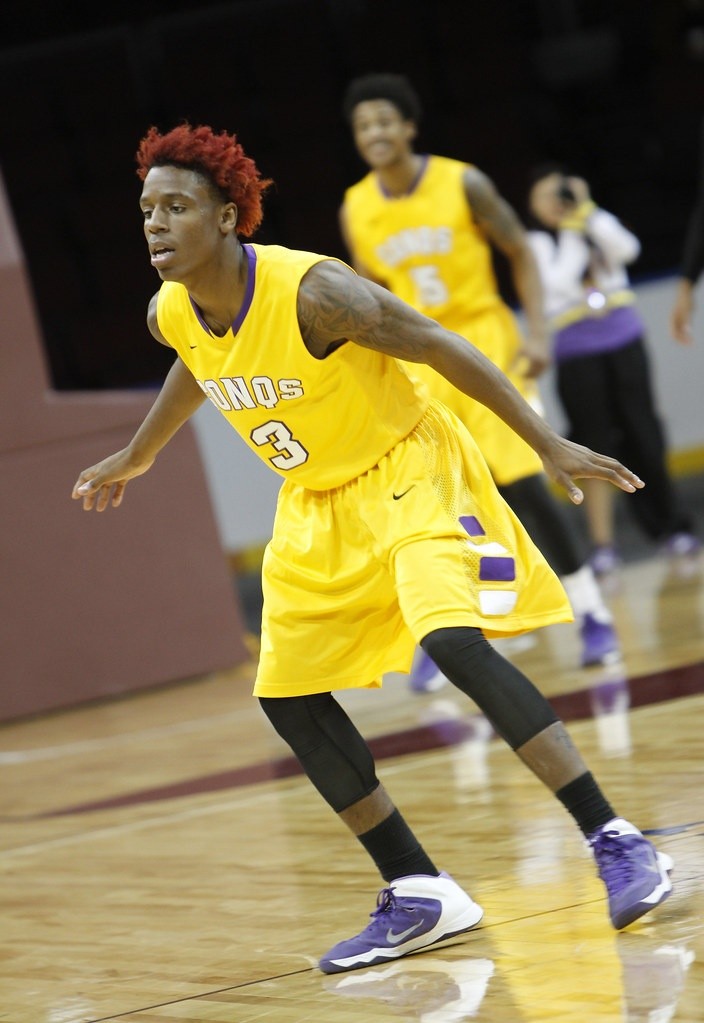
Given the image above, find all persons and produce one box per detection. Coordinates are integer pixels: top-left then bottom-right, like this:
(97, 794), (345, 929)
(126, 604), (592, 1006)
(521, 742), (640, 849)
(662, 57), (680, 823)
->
(525, 171), (696, 579)
(70, 122), (676, 976)
(338, 74), (621, 693)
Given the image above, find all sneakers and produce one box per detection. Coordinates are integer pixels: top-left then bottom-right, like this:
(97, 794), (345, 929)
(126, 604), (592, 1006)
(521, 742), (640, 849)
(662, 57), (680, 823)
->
(572, 603), (619, 670)
(595, 550), (618, 577)
(584, 676), (630, 758)
(666, 532), (704, 566)
(615, 932), (694, 1023)
(586, 818), (674, 930)
(321, 955), (495, 1023)
(411, 648), (447, 697)
(423, 701), (494, 782)
(319, 870), (484, 975)
